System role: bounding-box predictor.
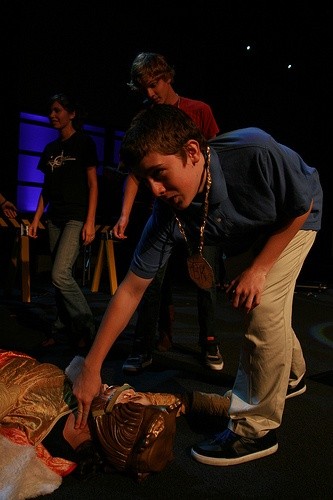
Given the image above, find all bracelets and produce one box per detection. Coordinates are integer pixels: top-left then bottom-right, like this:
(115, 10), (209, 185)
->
(0, 200), (7, 206)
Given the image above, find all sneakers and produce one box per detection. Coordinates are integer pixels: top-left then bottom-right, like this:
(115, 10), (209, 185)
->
(202, 345), (223, 370)
(190, 428), (278, 465)
(122, 352), (152, 372)
(285, 380), (306, 400)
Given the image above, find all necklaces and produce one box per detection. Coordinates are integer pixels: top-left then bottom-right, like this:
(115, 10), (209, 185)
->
(175, 93), (180, 110)
(167, 146), (215, 289)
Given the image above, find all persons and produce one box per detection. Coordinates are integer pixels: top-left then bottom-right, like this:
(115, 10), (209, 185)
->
(0, 348), (233, 500)
(29, 93), (98, 356)
(73, 104), (323, 466)
(112, 52), (224, 370)
(0, 194), (17, 218)
